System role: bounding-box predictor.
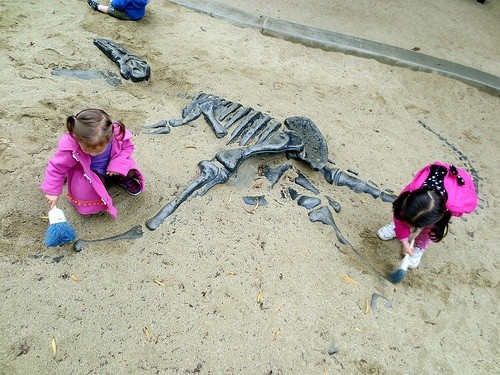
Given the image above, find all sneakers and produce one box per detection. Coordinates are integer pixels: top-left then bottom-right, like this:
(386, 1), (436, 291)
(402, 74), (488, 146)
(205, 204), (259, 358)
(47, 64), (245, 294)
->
(120, 179), (141, 195)
(377, 222), (396, 240)
(408, 239), (425, 268)
(87, 0), (100, 10)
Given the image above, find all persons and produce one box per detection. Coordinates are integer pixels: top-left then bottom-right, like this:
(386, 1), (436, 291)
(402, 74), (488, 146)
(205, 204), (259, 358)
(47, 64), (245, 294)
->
(86, 0), (149, 21)
(376, 161), (478, 286)
(40, 108), (144, 221)
